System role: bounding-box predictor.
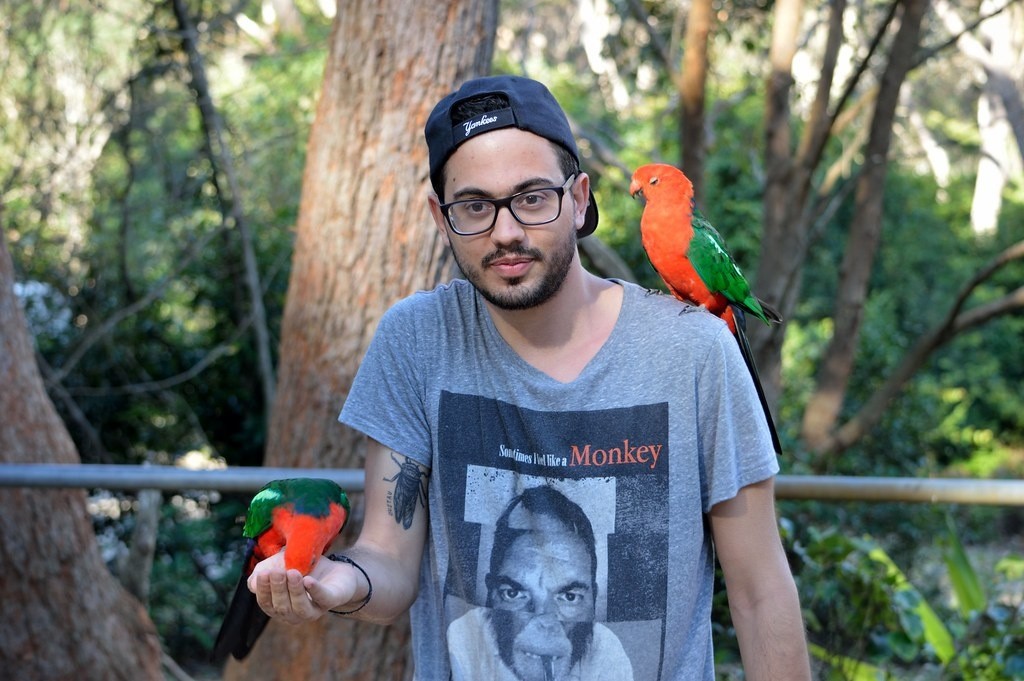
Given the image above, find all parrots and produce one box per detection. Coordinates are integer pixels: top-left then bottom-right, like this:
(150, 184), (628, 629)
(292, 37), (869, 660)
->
(207, 476), (351, 666)
(628, 162), (784, 454)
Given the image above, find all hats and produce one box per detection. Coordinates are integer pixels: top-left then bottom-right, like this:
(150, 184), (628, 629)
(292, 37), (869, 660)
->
(424, 74), (599, 238)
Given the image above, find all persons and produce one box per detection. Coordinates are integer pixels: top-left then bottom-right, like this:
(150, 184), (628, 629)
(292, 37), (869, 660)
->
(246, 74), (814, 681)
(482, 526), (599, 623)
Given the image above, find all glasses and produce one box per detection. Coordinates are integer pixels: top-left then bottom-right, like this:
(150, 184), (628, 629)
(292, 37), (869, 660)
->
(438, 173), (575, 235)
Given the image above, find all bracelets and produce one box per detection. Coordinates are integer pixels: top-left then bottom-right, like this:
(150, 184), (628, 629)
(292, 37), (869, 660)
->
(322, 553), (372, 615)
